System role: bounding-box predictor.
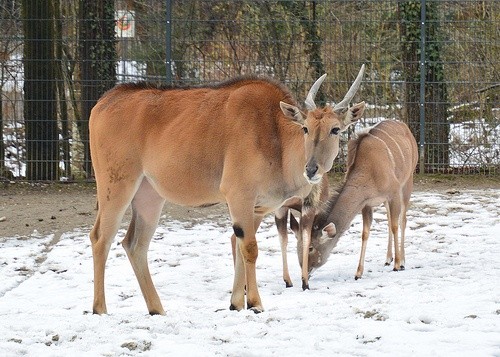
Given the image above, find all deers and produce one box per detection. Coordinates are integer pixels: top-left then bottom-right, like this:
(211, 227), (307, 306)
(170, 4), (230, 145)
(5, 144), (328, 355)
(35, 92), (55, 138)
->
(88, 63), (366, 314)
(230, 173), (327, 295)
(290, 119), (419, 280)
